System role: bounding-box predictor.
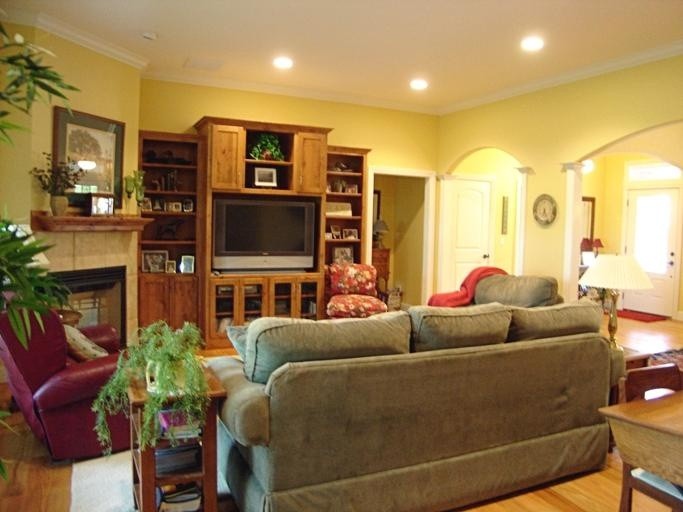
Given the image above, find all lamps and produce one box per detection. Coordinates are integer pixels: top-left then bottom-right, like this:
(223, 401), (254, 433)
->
(579, 255), (653, 348)
(581, 238), (592, 251)
(78, 143), (98, 170)
(592, 239), (604, 258)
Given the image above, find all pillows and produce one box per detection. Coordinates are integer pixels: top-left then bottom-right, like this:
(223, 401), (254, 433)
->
(474, 274), (558, 306)
(64, 323), (109, 362)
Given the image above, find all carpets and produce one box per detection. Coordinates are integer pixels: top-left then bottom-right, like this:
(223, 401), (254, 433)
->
(70, 450), (238, 512)
(649, 348), (683, 372)
(604, 310), (666, 322)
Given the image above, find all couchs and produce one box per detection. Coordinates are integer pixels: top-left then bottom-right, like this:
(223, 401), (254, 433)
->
(207, 333), (626, 512)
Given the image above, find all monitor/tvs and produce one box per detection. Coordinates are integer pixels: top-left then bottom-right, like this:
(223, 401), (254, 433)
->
(212, 197), (315, 274)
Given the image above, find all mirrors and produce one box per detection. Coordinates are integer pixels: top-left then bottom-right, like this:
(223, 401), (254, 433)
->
(374, 189), (381, 220)
(582, 195), (595, 249)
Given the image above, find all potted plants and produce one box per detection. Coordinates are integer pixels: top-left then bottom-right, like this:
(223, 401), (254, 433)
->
(92, 320), (212, 460)
(374, 220), (388, 248)
(249, 133), (284, 161)
(29, 152), (87, 216)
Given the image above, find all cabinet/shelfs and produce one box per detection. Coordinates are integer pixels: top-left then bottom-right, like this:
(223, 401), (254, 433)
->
(138, 129), (199, 272)
(287, 123), (334, 193)
(327, 144), (371, 267)
(373, 247), (390, 294)
(137, 271), (198, 333)
(126, 364), (227, 512)
(201, 278), (268, 352)
(193, 116), (246, 196)
(268, 273), (326, 320)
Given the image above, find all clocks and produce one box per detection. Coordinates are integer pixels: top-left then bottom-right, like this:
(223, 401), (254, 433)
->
(534, 195), (556, 225)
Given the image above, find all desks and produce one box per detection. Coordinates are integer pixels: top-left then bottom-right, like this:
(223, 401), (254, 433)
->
(598, 390), (683, 489)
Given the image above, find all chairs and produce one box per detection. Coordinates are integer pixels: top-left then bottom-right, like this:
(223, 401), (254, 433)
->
(0, 291), (131, 462)
(327, 262), (387, 318)
(618, 363), (683, 512)
(429, 267), (563, 308)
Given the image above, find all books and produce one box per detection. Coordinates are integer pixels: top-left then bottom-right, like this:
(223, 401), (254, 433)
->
(154, 410), (203, 511)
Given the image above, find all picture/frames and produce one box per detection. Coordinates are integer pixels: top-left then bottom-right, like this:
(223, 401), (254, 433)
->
(141, 250), (194, 275)
(51, 104), (125, 209)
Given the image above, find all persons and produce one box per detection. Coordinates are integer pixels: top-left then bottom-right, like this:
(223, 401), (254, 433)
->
(168, 265), (173, 273)
